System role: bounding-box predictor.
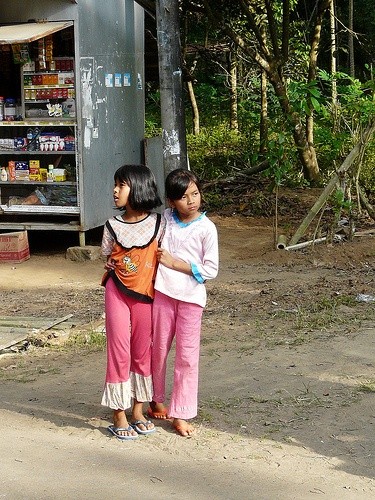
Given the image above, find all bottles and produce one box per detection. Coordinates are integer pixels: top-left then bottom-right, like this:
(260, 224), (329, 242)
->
(4, 99), (16, 121)
(48, 165), (53, 173)
(33, 127), (40, 151)
(0, 167), (7, 182)
(0, 97), (4, 121)
(27, 127), (33, 151)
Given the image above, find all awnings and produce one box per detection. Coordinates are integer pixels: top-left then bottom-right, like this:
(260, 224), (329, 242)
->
(0, 20), (73, 44)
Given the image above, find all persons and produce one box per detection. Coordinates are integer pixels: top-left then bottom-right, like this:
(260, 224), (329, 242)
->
(104, 169), (218, 439)
(101, 164), (166, 440)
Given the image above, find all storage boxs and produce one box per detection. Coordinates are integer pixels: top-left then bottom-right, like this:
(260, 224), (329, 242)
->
(0, 230), (30, 264)
(6, 159), (67, 183)
(23, 75), (76, 100)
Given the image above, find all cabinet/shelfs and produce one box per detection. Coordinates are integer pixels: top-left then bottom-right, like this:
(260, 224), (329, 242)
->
(0, 120), (81, 217)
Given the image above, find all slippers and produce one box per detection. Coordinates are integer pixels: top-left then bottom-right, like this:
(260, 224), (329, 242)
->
(147, 406), (172, 420)
(106, 424), (139, 440)
(128, 421), (156, 435)
(171, 421), (195, 438)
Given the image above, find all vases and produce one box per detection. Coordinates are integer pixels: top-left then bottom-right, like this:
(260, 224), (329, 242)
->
(20, 56), (76, 120)
